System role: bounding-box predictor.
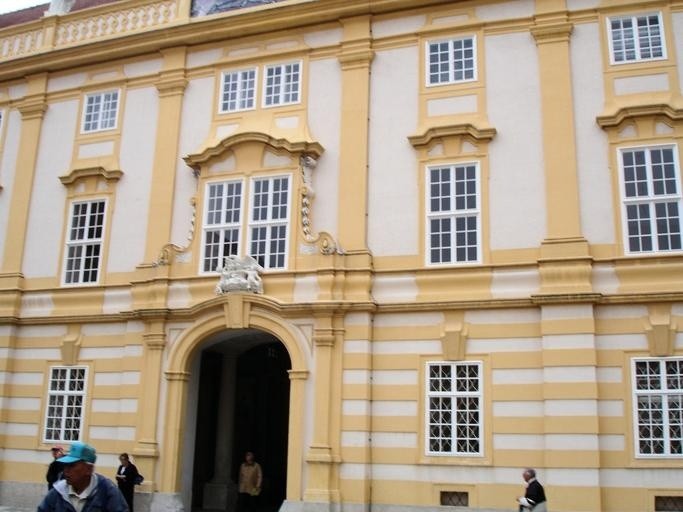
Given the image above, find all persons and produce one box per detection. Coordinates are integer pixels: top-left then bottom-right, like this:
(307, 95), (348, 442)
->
(238, 451), (263, 496)
(516, 469), (547, 512)
(46, 445), (66, 491)
(37, 441), (130, 512)
(115, 453), (138, 512)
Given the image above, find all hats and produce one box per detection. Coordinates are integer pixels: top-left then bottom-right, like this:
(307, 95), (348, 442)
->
(56, 442), (95, 464)
(52, 445), (63, 450)
